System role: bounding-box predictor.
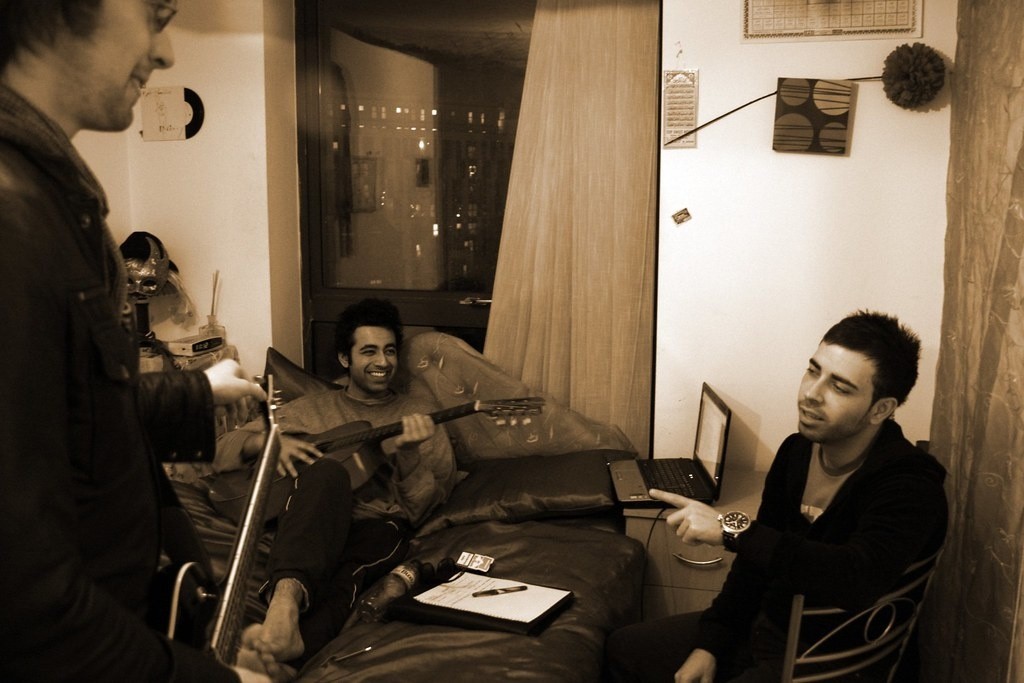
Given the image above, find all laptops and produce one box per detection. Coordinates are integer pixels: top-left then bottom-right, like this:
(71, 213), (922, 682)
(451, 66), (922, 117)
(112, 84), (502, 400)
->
(607, 381), (732, 508)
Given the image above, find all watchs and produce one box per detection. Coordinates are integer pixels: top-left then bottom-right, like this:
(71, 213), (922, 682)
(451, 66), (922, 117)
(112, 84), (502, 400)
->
(717, 509), (751, 554)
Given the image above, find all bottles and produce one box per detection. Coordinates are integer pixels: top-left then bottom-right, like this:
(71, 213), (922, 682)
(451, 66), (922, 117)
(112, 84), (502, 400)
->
(354, 558), (424, 625)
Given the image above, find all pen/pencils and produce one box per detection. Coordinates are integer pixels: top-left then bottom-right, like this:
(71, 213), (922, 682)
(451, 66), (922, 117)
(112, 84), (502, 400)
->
(473, 584), (529, 598)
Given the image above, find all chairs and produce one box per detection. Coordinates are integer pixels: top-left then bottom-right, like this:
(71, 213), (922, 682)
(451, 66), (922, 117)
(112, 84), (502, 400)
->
(781, 548), (946, 683)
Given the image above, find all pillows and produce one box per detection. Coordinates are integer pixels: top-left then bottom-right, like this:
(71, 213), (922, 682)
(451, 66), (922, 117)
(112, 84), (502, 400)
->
(266, 346), (346, 410)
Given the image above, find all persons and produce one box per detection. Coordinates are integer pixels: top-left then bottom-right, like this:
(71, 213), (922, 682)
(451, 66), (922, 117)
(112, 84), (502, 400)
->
(1, 0), (270, 683)
(599, 311), (948, 683)
(212, 297), (456, 683)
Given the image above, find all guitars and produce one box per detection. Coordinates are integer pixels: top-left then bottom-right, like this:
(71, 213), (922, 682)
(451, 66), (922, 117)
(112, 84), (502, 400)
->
(196, 393), (548, 537)
(120, 371), (286, 682)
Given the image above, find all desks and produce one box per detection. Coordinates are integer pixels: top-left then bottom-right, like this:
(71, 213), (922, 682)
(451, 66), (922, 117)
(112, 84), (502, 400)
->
(626, 459), (768, 614)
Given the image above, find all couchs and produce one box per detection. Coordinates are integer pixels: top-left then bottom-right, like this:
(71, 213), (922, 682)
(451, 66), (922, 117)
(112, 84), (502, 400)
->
(225, 330), (649, 683)
